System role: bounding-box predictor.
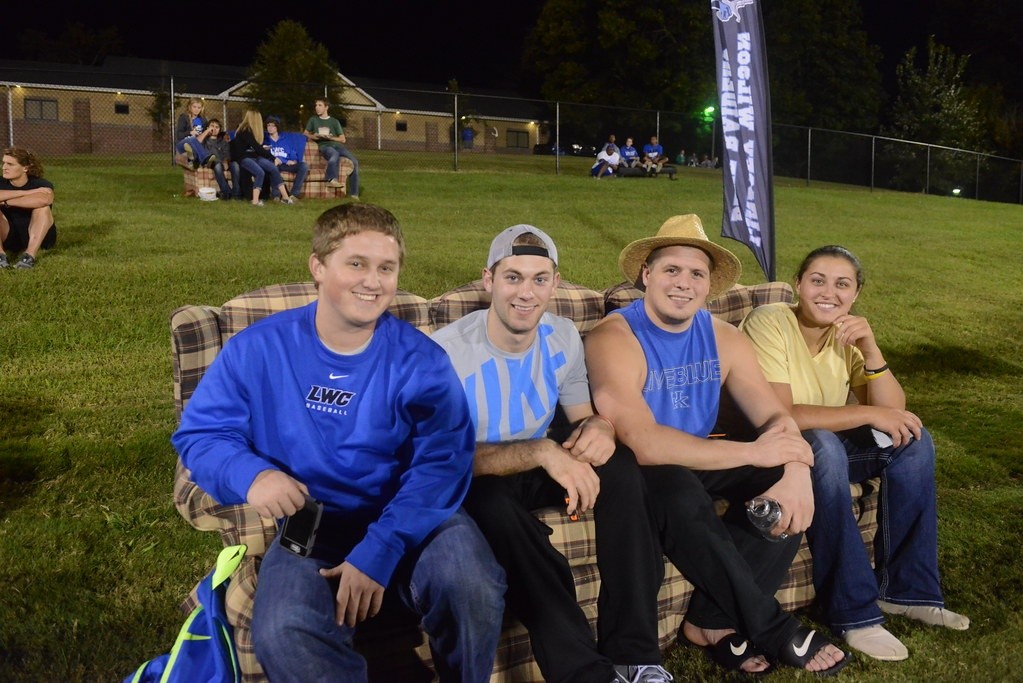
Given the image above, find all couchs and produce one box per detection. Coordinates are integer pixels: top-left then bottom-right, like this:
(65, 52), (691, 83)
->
(176, 142), (355, 198)
(168, 280), (886, 683)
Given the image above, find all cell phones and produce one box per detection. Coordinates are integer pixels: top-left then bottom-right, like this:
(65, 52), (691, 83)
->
(871, 429), (914, 448)
(278, 494), (324, 558)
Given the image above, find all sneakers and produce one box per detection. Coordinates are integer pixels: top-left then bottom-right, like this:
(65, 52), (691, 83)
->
(613, 664), (674, 683)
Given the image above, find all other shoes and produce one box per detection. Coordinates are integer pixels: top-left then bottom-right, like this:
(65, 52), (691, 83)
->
(842, 624), (909, 661)
(280, 198), (293, 205)
(183, 143), (195, 161)
(646, 162), (652, 172)
(253, 200), (264, 207)
(12, 253), (35, 269)
(631, 157), (640, 168)
(621, 158), (628, 168)
(351, 195), (361, 204)
(874, 598), (969, 630)
(0, 253), (10, 268)
(205, 154), (216, 168)
(656, 163), (663, 173)
(324, 178), (344, 188)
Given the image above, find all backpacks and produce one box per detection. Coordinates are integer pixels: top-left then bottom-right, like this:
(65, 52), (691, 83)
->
(122, 545), (247, 683)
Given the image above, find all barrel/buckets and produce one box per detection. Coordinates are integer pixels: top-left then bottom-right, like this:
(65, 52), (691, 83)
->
(198, 186), (216, 200)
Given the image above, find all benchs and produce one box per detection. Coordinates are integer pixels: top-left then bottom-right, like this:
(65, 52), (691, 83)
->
(616, 164), (678, 180)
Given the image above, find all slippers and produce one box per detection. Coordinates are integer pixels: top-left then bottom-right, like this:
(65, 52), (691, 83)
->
(677, 618), (780, 676)
(778, 624), (852, 675)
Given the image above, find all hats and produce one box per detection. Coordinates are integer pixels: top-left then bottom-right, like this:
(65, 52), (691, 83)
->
(618, 213), (742, 304)
(487, 223), (558, 269)
(606, 144), (615, 150)
(265, 112), (281, 124)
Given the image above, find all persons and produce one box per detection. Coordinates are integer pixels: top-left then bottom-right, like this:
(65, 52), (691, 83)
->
(591, 143), (620, 180)
(177, 98), (216, 169)
(229, 108), (294, 206)
(643, 135), (668, 165)
(582, 211), (848, 672)
(620, 137), (662, 173)
(263, 114), (307, 203)
(195, 117), (244, 201)
(739, 244), (971, 661)
(687, 153), (699, 168)
(171, 200), (508, 683)
(701, 154), (719, 169)
(676, 149), (686, 165)
(303, 96), (361, 201)
(601, 134), (638, 168)
(421, 225), (673, 683)
(0, 147), (57, 269)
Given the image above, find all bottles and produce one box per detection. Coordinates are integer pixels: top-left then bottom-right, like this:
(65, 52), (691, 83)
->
(746, 496), (790, 543)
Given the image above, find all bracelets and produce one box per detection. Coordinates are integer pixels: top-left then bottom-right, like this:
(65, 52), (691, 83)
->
(3, 200), (10, 208)
(591, 415), (615, 432)
(862, 363), (889, 380)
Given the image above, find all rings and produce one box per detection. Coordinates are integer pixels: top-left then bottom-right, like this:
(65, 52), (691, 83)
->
(779, 532), (790, 540)
